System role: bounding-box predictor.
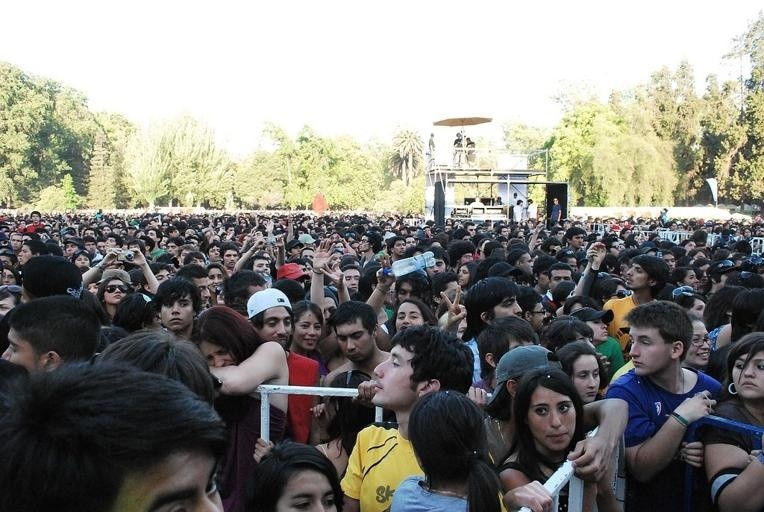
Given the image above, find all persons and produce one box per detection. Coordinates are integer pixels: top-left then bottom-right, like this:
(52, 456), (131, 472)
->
(1, 193), (764, 512)
(465, 138), (475, 168)
(453, 132), (463, 167)
(428, 133), (435, 166)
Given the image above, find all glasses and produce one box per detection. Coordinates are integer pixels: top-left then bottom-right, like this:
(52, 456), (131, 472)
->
(106, 285), (128, 293)
(362, 239), (371, 243)
(692, 338), (715, 347)
(140, 292), (152, 308)
(532, 308), (546, 316)
(612, 290), (632, 298)
(0, 285), (21, 293)
(611, 245), (621, 249)
(346, 232), (355, 237)
(672, 286), (694, 299)
(74, 250), (89, 255)
(712, 256), (764, 282)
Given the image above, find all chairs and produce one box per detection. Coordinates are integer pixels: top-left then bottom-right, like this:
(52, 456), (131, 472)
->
(450, 208), (473, 218)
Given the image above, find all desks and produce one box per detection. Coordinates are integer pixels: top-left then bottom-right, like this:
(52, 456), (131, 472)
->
(452, 148), (478, 169)
(495, 154), (528, 171)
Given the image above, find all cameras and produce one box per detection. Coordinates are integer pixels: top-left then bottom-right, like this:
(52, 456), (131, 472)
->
(117, 251), (135, 261)
(266, 238), (277, 244)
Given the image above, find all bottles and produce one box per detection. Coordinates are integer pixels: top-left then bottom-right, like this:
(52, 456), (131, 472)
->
(382, 250), (437, 279)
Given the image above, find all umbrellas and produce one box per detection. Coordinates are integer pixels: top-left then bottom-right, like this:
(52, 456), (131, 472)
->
(433, 117), (492, 137)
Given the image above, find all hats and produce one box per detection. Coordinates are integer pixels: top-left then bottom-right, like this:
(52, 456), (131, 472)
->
(487, 345), (563, 406)
(298, 234), (316, 244)
(285, 240), (304, 251)
(706, 260), (742, 275)
(488, 262), (523, 277)
(22, 255), (83, 299)
(569, 307), (614, 323)
(277, 264), (310, 280)
(247, 288), (293, 319)
(641, 247), (658, 254)
(96, 269), (131, 285)
